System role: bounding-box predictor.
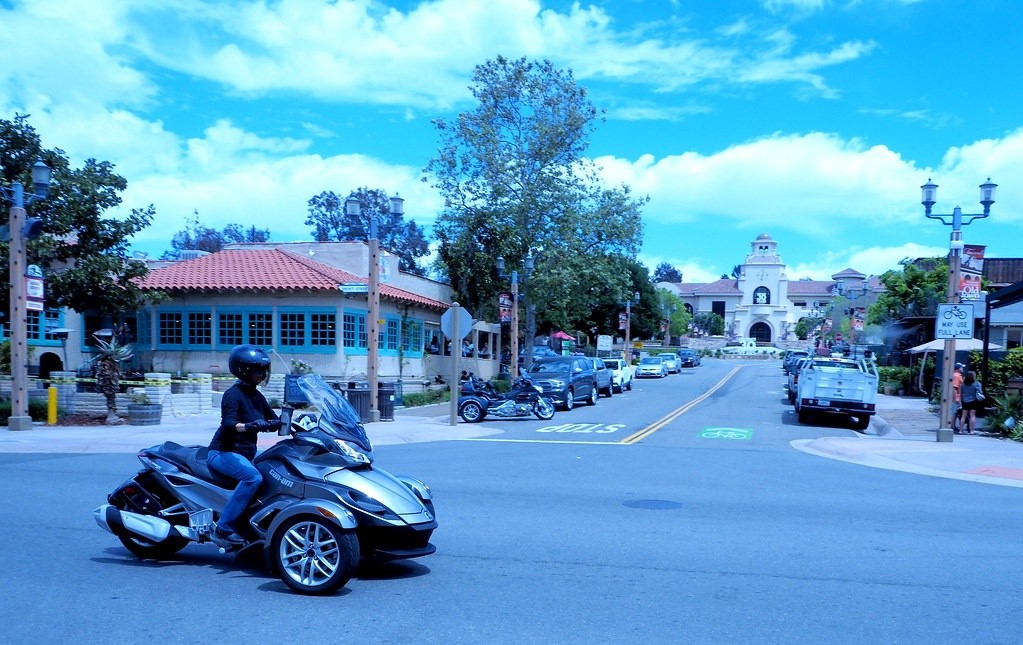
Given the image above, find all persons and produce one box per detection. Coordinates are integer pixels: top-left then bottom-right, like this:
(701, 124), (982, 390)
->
(958, 371), (983, 435)
(951, 363), (965, 432)
(430, 336), (489, 358)
(208, 344), (280, 545)
(461, 371), (474, 380)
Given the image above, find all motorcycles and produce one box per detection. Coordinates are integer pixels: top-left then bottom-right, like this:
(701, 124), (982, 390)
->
(458, 367), (555, 423)
(92, 373), (438, 595)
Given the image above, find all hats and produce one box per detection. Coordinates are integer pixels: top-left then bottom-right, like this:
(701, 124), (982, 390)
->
(954, 363), (966, 370)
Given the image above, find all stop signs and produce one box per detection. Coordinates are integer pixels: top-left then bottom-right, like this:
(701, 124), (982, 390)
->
(836, 333), (842, 341)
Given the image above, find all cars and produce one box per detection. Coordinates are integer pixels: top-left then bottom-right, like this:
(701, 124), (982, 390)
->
(512, 355), (600, 409)
(657, 353), (681, 373)
(518, 345), (552, 371)
(602, 359), (633, 393)
(676, 349), (701, 368)
(780, 349), (812, 402)
(635, 357), (670, 378)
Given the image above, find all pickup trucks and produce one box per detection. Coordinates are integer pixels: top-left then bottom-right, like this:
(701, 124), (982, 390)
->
(790, 357), (879, 429)
(573, 357), (613, 397)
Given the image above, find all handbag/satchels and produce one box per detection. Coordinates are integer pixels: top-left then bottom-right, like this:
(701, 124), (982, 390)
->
(976, 382), (985, 401)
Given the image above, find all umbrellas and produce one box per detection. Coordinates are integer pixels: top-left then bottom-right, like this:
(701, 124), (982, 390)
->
(550, 331), (576, 351)
(903, 338), (1002, 364)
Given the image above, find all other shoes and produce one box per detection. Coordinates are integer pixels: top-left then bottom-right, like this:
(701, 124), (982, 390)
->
(970, 432), (975, 435)
(958, 431), (962, 434)
(951, 427), (959, 431)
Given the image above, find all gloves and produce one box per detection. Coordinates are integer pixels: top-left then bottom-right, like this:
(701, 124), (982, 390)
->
(245, 419), (270, 433)
(295, 414), (317, 423)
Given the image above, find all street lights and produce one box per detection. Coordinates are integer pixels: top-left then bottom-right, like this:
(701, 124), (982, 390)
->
(345, 192), (405, 422)
(496, 255), (535, 385)
(837, 278), (869, 346)
(617, 291), (641, 365)
(49, 328), (77, 372)
(1, 156), (53, 431)
(920, 177), (998, 442)
(660, 303), (677, 349)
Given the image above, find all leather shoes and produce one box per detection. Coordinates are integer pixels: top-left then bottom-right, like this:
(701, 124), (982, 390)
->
(215, 525), (244, 544)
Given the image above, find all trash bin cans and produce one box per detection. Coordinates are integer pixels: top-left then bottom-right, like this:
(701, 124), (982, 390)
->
(632, 350), (640, 358)
(347, 381), (371, 423)
(378, 382), (397, 421)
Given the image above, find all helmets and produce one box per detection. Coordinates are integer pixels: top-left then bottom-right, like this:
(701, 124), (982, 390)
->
(229, 344), (272, 388)
